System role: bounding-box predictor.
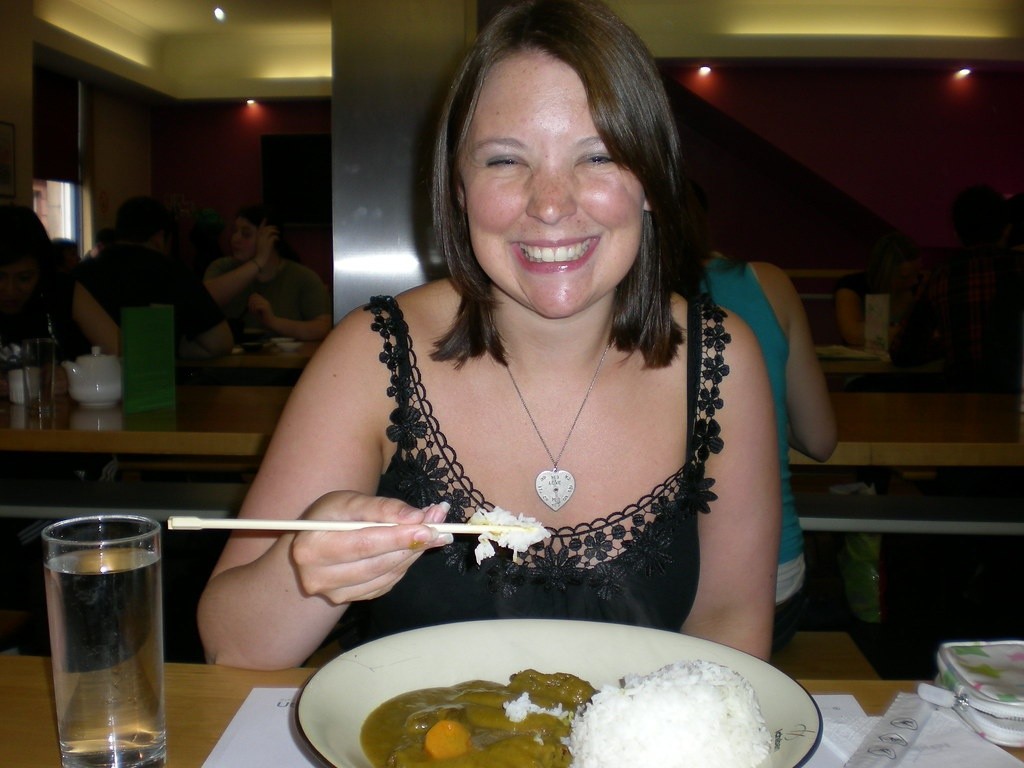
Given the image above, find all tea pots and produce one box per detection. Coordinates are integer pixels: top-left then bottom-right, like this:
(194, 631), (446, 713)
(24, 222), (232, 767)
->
(61, 346), (123, 407)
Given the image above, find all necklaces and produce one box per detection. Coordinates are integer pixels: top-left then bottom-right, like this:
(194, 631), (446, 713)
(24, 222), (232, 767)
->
(499, 344), (611, 511)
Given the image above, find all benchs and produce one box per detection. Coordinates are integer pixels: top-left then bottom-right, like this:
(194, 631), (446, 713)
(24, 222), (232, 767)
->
(296, 631), (875, 683)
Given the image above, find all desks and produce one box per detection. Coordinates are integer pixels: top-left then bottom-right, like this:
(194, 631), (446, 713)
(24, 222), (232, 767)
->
(0, 264), (1024, 768)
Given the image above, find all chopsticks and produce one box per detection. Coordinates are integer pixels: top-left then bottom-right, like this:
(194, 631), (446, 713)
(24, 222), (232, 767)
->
(167, 515), (536, 536)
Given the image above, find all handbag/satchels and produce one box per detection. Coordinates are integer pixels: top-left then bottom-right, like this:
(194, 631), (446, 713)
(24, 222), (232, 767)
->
(918, 639), (1024, 748)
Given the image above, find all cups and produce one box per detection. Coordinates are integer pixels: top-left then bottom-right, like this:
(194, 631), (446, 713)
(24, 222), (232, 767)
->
(9, 367), (41, 405)
(22, 338), (56, 409)
(41, 514), (166, 768)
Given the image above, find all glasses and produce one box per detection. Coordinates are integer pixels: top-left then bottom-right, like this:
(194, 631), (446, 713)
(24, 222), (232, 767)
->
(233, 225), (258, 239)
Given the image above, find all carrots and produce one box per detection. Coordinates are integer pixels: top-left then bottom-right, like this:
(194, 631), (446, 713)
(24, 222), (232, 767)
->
(425, 720), (473, 761)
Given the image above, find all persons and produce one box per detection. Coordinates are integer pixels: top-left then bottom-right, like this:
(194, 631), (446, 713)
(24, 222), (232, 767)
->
(195, 0), (778, 668)
(671, 173), (838, 655)
(0, 195), (331, 656)
(833, 185), (1024, 394)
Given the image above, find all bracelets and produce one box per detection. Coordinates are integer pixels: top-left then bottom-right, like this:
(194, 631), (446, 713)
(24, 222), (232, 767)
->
(254, 259), (261, 272)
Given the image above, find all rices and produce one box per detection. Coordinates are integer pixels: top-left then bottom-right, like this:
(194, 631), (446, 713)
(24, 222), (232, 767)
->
(466, 505), (552, 566)
(502, 659), (773, 767)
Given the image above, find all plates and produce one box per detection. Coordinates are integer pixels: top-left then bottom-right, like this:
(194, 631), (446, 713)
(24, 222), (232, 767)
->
(295, 618), (823, 768)
(276, 342), (303, 352)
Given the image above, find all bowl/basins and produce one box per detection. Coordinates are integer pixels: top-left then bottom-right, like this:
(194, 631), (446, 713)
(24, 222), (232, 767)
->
(271, 337), (296, 345)
